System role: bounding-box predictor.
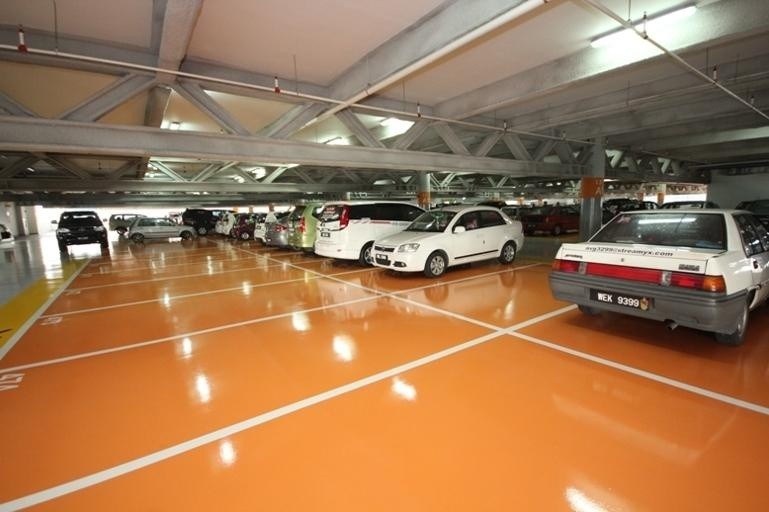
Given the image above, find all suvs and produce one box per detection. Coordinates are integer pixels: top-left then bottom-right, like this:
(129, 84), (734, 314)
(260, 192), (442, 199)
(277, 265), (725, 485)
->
(286, 202), (327, 255)
(313, 200), (438, 266)
(108, 213), (148, 236)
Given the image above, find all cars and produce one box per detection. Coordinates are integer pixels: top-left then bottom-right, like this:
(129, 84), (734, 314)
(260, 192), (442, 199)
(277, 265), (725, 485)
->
(369, 203), (524, 279)
(123, 217), (198, 243)
(51, 210), (109, 252)
(480, 196), (769, 237)
(167, 200), (287, 248)
(0, 223), (14, 242)
(549, 209), (768, 346)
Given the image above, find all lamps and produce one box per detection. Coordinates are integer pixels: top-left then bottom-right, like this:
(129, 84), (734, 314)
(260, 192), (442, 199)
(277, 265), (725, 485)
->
(590, 6), (697, 48)
(170, 115), (396, 181)
